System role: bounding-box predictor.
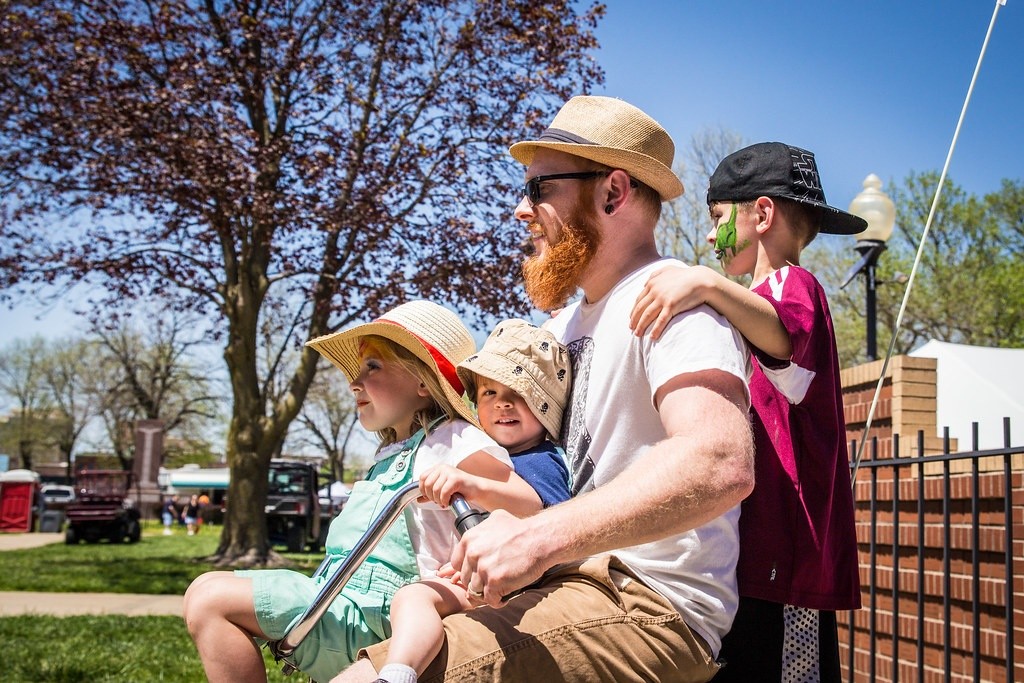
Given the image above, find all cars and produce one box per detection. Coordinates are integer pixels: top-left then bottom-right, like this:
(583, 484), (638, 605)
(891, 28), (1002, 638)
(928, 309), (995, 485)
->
(39, 486), (75, 515)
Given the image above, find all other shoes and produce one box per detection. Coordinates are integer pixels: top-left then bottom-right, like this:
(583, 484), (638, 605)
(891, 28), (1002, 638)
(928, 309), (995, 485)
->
(188, 530), (193, 535)
(164, 530), (171, 535)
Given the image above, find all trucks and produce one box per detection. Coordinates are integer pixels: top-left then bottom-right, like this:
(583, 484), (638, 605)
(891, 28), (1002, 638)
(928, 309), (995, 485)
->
(265, 459), (345, 553)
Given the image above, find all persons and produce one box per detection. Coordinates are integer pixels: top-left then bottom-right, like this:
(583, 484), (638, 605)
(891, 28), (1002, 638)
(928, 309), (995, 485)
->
(181, 492), (202, 536)
(183, 95), (868, 683)
(162, 495), (179, 535)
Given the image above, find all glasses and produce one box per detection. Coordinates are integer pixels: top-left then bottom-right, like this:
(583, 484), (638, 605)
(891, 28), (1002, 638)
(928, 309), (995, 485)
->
(521, 171), (637, 206)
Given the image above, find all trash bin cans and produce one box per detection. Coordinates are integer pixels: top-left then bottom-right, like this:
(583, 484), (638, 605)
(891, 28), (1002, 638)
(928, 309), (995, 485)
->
(38, 484), (76, 533)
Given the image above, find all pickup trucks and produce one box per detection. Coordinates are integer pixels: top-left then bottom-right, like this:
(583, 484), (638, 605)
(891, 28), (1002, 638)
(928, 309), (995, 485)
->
(65, 470), (143, 546)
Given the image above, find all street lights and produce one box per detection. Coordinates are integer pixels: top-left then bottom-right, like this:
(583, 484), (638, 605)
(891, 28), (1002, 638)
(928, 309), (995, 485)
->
(852, 174), (898, 364)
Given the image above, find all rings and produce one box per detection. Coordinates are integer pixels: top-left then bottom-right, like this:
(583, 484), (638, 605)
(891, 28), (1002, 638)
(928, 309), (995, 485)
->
(468, 581), (484, 597)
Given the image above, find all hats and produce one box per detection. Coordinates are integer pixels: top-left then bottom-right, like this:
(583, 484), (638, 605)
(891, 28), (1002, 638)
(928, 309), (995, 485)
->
(454, 318), (572, 441)
(508, 95), (685, 200)
(706, 142), (869, 235)
(304, 300), (486, 434)
